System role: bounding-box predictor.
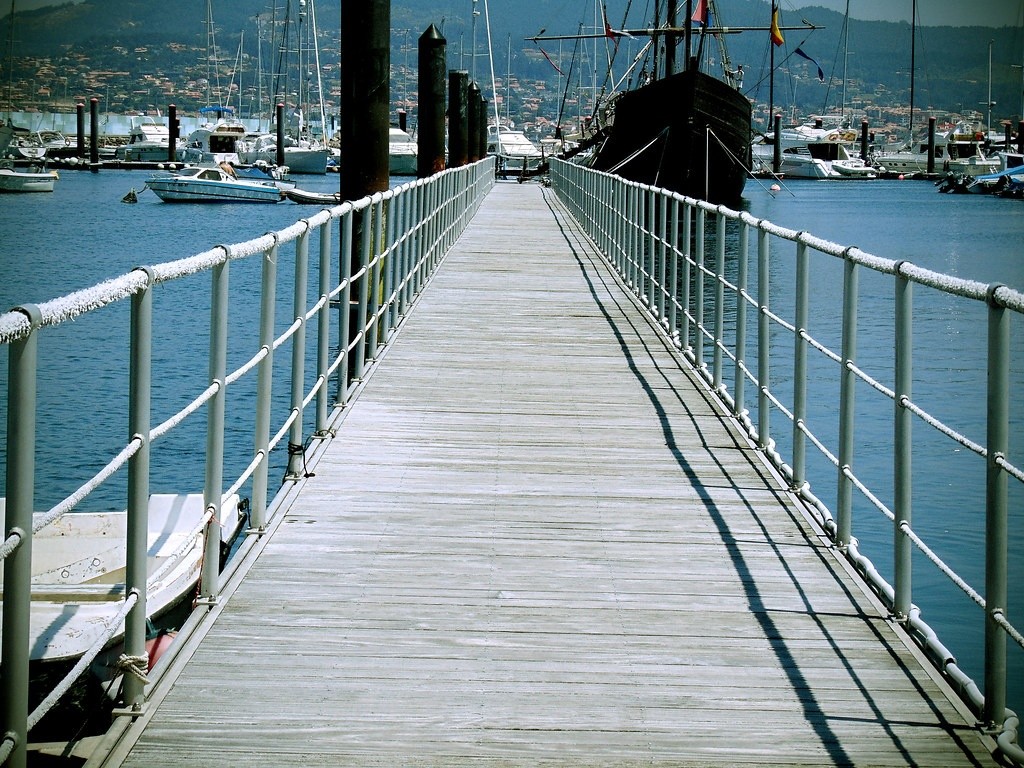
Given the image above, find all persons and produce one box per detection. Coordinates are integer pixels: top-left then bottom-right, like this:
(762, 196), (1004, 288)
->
(728, 64), (745, 92)
(273, 164), (283, 180)
(220, 160), (238, 179)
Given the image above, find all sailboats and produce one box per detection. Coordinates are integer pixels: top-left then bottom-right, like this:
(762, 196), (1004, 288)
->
(0, 0), (341, 174)
(495, 0), (826, 221)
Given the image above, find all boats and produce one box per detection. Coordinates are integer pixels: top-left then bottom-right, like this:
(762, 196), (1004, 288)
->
(121, 163), (340, 204)
(749, 124), (1024, 199)
(389, 129), (418, 175)
(0, 168), (55, 192)
(487, 125), (542, 167)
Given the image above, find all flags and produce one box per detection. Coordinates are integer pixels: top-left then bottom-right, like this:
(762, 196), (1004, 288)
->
(691, 0), (714, 28)
(605, 15), (617, 43)
(770, 3), (784, 47)
